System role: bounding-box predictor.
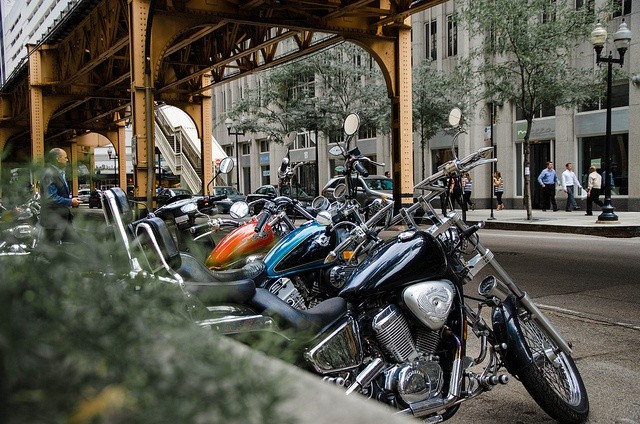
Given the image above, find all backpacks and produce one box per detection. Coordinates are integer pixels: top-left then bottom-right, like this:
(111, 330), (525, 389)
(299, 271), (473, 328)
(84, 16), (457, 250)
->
(458, 181), (461, 194)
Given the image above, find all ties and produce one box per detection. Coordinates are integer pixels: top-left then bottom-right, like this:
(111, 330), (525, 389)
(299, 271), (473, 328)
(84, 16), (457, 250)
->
(61, 172), (69, 193)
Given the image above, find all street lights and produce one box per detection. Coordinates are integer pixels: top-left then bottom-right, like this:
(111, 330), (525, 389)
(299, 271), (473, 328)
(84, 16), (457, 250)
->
(107, 145), (118, 187)
(590, 17), (633, 224)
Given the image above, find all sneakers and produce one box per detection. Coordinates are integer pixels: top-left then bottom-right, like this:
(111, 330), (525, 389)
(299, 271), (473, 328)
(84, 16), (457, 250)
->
(471, 203), (475, 211)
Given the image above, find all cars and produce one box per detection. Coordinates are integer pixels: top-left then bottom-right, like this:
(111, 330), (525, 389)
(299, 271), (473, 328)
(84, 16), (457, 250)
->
(156, 187), (193, 210)
(252, 184), (314, 216)
(197, 186), (246, 214)
(88, 189), (102, 208)
(69, 188), (102, 203)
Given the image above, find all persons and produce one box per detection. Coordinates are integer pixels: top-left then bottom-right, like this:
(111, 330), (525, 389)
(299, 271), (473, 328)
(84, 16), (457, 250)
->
(602, 165), (615, 194)
(462, 172), (475, 211)
(381, 171), (393, 189)
(448, 170), (463, 212)
(40, 147), (82, 242)
(537, 161), (562, 211)
(493, 171), (504, 210)
(562, 162), (583, 211)
(585, 165), (604, 215)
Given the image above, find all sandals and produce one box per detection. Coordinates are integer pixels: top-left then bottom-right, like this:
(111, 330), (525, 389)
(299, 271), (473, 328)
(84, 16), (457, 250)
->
(497, 206), (502, 210)
(501, 204), (504, 208)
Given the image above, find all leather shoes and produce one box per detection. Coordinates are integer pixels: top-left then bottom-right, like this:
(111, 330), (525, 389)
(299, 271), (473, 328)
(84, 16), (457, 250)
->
(566, 209), (572, 212)
(574, 206), (581, 210)
(585, 213), (592, 216)
(542, 208), (546, 211)
(553, 208), (560, 211)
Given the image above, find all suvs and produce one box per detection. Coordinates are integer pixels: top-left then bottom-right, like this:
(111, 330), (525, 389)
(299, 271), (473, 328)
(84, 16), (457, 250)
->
(320, 174), (426, 225)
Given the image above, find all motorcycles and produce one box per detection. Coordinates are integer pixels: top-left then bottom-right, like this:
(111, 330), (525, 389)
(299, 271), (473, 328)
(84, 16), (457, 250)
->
(126, 105), (592, 423)
(0, 179), (41, 257)
(97, 111), (398, 315)
(132, 129), (305, 273)
(130, 157), (235, 252)
